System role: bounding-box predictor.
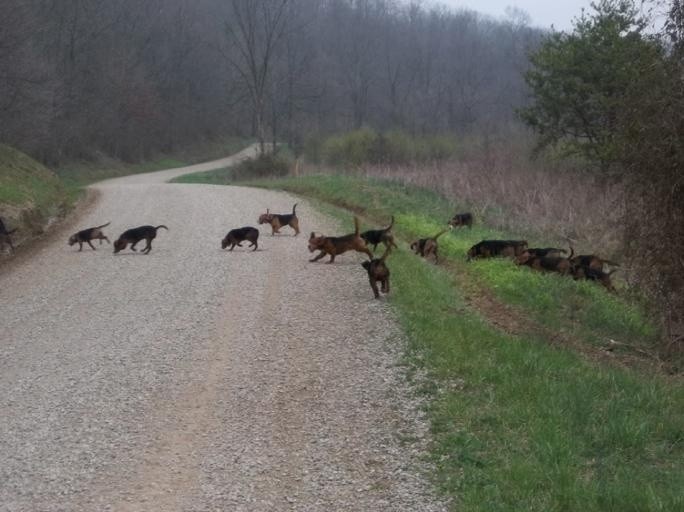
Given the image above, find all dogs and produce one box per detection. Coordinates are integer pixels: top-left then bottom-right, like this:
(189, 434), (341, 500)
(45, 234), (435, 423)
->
(447, 213), (473, 233)
(114, 224), (169, 256)
(307, 215), (372, 264)
(466, 239), (619, 297)
(220, 226), (259, 251)
(257, 203), (300, 238)
(361, 242), (392, 299)
(0, 221), (18, 256)
(68, 221), (114, 251)
(360, 216), (398, 257)
(410, 229), (448, 266)
